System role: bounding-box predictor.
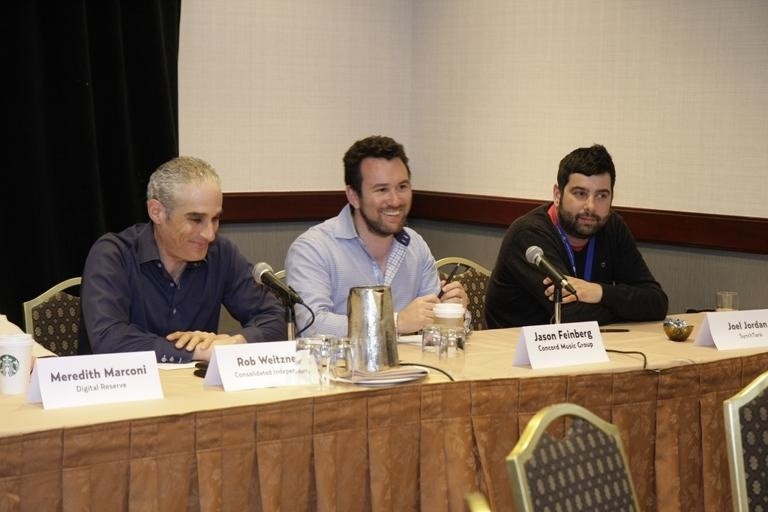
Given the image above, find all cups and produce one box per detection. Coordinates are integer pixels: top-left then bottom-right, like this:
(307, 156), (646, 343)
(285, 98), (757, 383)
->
(0, 335), (33, 398)
(716, 292), (740, 311)
(298, 332), (354, 384)
(422, 324), (468, 361)
(434, 301), (467, 327)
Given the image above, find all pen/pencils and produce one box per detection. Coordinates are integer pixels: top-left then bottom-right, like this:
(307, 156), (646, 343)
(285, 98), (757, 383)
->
(437, 262), (461, 299)
(600, 329), (631, 332)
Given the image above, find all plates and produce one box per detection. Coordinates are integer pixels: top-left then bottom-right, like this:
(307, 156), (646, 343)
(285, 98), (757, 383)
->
(330, 366), (432, 390)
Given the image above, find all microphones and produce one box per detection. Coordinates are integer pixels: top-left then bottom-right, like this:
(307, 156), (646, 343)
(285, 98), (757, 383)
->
(525, 245), (577, 295)
(251, 262), (304, 305)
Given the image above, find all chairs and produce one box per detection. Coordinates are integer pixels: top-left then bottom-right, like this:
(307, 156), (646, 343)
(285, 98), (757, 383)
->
(22, 274), (91, 359)
(436, 254), (494, 332)
(723, 367), (768, 511)
(502, 399), (642, 511)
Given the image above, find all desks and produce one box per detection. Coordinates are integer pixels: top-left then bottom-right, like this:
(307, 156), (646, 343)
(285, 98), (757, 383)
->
(1, 306), (768, 511)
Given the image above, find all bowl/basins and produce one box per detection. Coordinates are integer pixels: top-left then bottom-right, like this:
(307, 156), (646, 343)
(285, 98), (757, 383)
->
(662, 318), (693, 342)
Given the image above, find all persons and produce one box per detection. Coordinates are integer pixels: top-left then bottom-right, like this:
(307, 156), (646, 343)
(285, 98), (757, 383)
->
(484, 145), (668, 329)
(285, 136), (471, 339)
(78, 157), (287, 362)
(1, 314), (59, 372)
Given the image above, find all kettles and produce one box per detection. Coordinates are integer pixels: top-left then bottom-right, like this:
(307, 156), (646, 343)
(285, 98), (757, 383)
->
(348, 285), (401, 371)
